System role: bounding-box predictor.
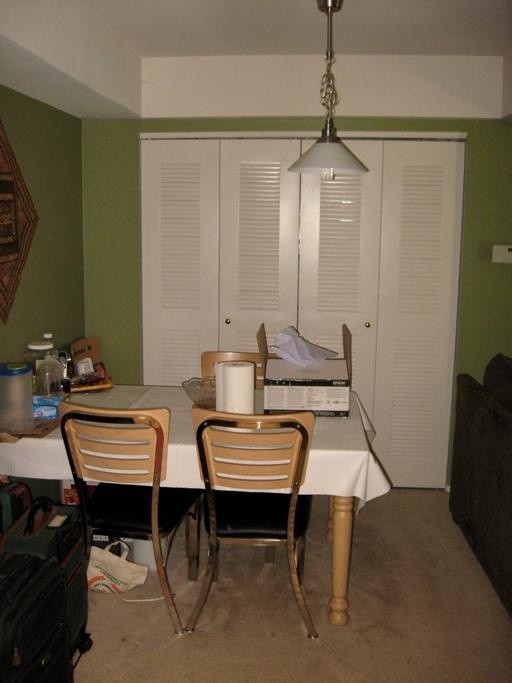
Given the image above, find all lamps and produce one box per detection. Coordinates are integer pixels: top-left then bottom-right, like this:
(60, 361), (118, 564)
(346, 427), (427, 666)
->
(286, 0), (370, 184)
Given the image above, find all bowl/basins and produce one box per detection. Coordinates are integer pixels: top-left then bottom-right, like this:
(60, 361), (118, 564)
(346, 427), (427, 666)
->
(181, 374), (217, 409)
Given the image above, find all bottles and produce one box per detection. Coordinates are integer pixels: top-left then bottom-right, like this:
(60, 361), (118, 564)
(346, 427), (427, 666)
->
(23, 331), (61, 399)
(0, 361), (33, 433)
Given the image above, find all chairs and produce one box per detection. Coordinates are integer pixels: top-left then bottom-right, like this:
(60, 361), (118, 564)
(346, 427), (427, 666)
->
(56, 401), (183, 650)
(70, 334), (104, 381)
(200, 350), (285, 394)
(186, 408), (318, 643)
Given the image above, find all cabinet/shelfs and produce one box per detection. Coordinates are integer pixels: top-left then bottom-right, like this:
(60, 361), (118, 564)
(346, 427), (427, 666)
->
(138, 135), (298, 385)
(298, 131), (467, 493)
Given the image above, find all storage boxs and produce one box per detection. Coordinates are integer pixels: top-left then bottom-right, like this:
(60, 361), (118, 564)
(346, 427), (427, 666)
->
(257, 322), (354, 421)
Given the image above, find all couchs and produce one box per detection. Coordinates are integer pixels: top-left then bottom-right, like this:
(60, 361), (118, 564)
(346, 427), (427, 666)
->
(448, 352), (512, 617)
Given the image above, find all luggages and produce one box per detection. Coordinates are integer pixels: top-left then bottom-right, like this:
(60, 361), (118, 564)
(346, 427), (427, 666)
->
(1, 482), (93, 682)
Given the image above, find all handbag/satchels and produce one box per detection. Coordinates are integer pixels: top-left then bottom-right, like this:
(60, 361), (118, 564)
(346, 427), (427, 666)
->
(89, 538), (147, 595)
